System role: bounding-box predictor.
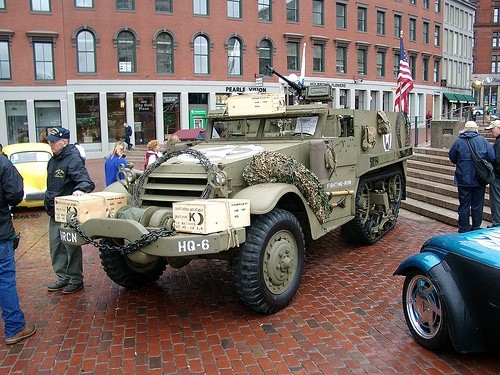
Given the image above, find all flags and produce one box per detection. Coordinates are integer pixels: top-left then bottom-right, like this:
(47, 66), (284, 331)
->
(394, 35), (415, 115)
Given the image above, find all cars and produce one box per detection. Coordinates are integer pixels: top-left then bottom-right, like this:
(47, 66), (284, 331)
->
(392, 226), (500, 357)
(472, 103), (496, 116)
(2, 142), (53, 209)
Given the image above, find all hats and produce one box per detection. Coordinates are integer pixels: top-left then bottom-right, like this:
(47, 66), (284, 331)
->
(465, 121), (477, 128)
(485, 119), (500, 130)
(44, 126), (70, 141)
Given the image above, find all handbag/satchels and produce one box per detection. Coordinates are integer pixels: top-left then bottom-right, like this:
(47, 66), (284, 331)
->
(474, 158), (495, 185)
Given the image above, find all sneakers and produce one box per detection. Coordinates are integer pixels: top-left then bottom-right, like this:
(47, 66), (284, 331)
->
(4, 324), (37, 345)
(47, 280), (68, 291)
(62, 282), (84, 293)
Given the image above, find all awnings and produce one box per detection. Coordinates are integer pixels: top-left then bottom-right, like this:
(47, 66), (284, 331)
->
(453, 94), (468, 104)
(444, 93), (458, 103)
(463, 95), (476, 104)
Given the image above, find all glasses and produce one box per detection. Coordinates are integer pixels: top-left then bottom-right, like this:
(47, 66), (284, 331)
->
(49, 141), (60, 144)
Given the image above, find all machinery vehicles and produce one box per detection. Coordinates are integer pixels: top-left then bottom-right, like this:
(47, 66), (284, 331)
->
(54, 64), (413, 316)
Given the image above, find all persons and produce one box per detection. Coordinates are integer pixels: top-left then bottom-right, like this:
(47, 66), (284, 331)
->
(104, 141), (127, 188)
(0, 143), (38, 344)
(144, 140), (160, 172)
(484, 119), (500, 229)
(44, 126), (96, 294)
(447, 121), (496, 233)
(123, 123), (133, 150)
(426, 110), (432, 128)
(168, 135), (180, 141)
